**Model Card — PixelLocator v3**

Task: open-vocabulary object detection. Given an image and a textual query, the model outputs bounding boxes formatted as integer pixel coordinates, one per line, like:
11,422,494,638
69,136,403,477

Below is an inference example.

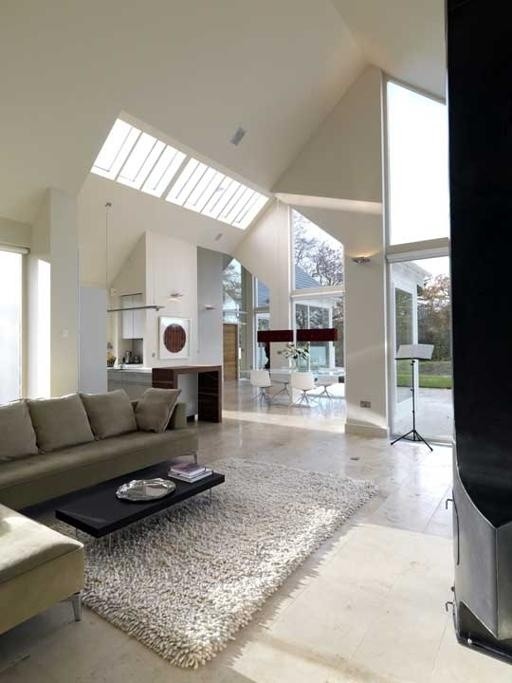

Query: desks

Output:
152,364,222,423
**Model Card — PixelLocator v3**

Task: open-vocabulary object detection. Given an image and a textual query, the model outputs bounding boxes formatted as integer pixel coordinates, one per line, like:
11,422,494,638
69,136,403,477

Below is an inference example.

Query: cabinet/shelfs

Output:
122,292,144,340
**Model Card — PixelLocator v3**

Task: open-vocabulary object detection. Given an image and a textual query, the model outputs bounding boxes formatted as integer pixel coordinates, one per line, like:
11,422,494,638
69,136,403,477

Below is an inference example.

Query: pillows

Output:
24,392,95,454
135,387,182,436
0,400,40,463
78,389,138,442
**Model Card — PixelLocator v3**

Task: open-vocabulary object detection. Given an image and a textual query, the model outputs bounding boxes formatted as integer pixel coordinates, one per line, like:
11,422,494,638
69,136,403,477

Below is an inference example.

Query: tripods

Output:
389,360,435,455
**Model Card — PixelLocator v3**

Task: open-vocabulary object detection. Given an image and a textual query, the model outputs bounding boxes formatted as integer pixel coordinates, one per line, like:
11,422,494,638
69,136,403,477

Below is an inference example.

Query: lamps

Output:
351,256,372,264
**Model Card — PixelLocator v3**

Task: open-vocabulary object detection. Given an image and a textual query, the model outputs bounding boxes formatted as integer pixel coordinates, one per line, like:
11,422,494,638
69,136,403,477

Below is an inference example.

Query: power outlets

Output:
360,400,371,409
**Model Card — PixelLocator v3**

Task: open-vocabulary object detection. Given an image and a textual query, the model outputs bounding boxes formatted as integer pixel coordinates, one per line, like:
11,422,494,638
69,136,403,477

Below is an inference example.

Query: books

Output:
168,461,214,483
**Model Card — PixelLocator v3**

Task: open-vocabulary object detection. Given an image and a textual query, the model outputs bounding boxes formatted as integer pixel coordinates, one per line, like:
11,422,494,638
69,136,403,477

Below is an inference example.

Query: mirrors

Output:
158,316,190,360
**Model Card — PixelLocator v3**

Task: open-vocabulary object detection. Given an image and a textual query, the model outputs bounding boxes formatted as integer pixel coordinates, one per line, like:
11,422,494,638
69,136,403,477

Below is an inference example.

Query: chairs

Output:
288,372,322,414
249,369,273,409
270,369,291,401
313,373,339,406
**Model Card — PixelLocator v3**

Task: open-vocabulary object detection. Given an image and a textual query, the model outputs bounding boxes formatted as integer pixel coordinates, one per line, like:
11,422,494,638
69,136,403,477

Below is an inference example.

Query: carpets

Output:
46,456,380,669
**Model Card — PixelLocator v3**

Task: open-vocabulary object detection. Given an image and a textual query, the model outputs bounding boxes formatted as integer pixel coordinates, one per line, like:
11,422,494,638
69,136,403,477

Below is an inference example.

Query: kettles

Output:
125,351,133,364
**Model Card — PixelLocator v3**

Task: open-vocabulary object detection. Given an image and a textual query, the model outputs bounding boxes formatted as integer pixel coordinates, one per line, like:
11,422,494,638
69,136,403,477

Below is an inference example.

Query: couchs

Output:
0,398,198,512
0,503,85,633
